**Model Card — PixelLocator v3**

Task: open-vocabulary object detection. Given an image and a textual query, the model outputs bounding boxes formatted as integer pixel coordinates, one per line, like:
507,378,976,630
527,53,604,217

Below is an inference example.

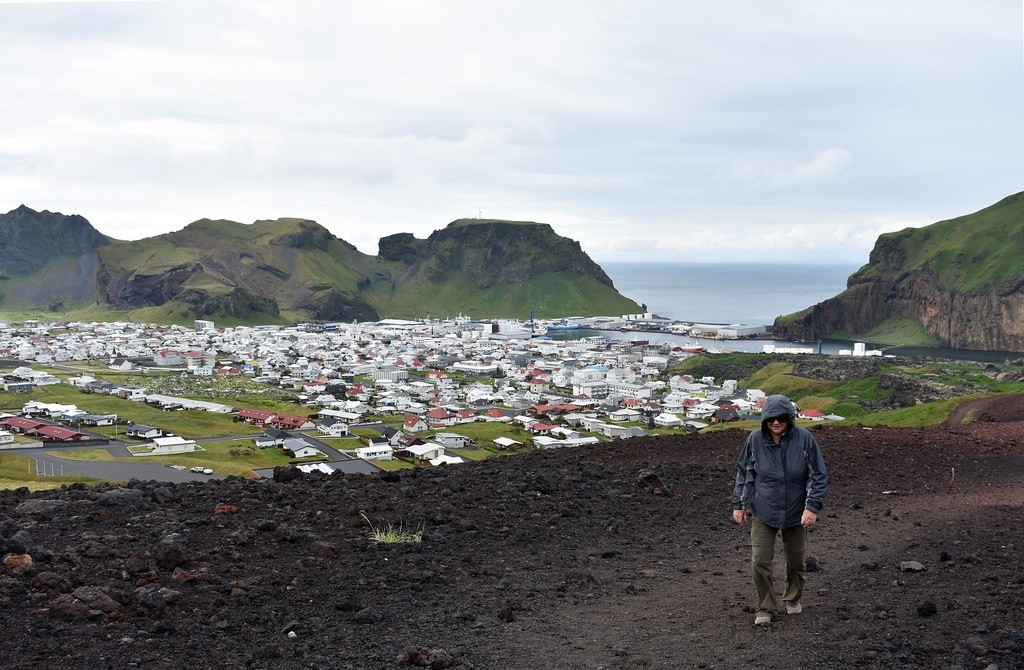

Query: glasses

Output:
765,415,790,424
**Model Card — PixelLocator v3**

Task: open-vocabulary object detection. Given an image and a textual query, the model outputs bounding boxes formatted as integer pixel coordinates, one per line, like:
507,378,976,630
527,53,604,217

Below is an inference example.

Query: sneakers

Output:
786,602,802,614
754,615,771,624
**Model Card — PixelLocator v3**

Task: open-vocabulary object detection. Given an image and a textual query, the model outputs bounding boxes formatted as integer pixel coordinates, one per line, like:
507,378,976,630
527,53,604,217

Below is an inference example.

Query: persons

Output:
732,393,829,625
